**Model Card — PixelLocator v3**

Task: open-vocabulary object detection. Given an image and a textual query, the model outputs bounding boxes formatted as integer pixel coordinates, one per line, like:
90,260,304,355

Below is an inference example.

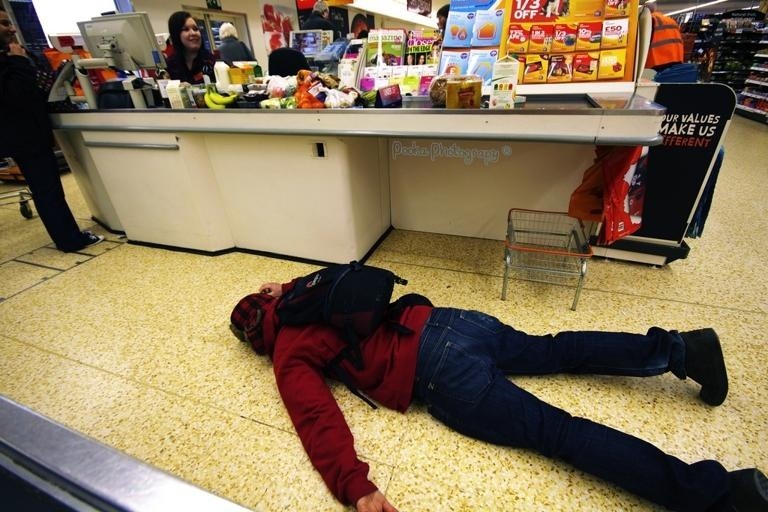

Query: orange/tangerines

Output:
294,84,325,108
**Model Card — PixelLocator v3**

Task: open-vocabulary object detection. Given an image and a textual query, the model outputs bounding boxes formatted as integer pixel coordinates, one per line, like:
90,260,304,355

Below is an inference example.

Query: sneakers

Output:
81,230,104,248
722,468,768,511
679,328,728,405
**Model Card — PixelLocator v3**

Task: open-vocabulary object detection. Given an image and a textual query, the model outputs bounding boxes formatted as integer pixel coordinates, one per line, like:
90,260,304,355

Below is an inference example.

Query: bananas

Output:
204,84,237,109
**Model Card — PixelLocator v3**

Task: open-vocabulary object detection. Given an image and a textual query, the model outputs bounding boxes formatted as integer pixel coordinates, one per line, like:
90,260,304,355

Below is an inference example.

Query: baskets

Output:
506,208,594,292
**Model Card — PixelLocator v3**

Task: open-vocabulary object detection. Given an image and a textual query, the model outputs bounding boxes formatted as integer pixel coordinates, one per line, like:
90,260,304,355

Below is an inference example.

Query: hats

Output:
231,293,276,356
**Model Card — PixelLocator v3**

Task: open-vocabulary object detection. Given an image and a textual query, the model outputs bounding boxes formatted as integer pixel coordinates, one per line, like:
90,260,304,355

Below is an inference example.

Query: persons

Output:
214,22,258,63
230,261,768,512
166,10,218,84
644,0,684,70
302,0,340,44
1,8,106,248
436,3,450,39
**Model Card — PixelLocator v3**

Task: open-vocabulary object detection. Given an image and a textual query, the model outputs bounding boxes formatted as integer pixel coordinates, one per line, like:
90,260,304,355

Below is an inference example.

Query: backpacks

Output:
269,261,394,363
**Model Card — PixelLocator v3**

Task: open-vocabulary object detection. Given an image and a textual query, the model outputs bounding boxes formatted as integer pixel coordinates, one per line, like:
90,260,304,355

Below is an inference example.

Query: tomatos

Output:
271,85,284,98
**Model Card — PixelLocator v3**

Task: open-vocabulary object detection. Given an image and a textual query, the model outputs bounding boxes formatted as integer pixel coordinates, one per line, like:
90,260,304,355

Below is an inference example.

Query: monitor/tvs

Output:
76,11,168,71
289,29,323,58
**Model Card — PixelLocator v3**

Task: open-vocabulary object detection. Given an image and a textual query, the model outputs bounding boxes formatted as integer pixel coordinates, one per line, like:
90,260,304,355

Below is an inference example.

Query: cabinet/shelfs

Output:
674,9,768,125
